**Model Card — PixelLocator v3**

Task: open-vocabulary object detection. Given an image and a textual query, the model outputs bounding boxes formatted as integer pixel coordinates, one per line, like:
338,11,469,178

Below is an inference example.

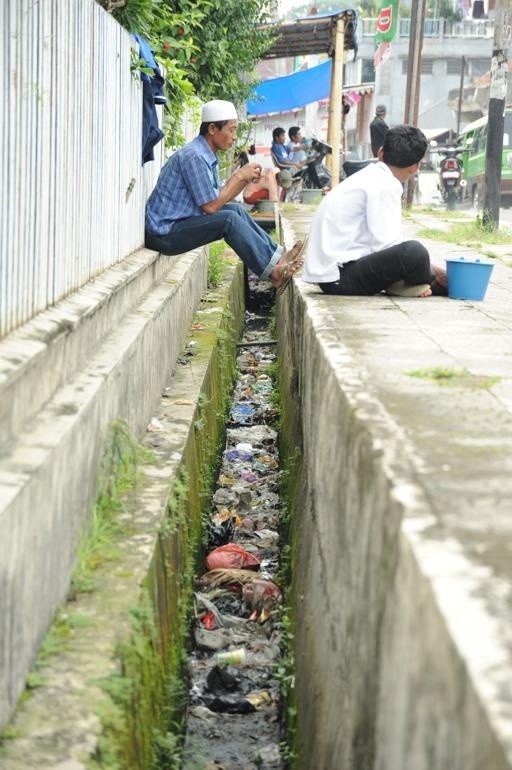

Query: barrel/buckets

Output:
301,188,323,205
255,200,276,214
446,257,495,301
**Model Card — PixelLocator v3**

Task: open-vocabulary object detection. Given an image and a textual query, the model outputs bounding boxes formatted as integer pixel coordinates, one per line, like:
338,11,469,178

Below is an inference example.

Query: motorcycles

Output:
432,143,470,210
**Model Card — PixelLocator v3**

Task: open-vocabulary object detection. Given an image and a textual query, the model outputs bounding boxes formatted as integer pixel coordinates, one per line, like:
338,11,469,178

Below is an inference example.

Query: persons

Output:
370,104,390,158
300,124,447,298
272,126,331,192
145,99,308,296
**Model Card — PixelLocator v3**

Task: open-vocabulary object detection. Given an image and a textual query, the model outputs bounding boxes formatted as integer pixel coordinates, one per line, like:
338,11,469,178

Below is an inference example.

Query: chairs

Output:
272,152,309,204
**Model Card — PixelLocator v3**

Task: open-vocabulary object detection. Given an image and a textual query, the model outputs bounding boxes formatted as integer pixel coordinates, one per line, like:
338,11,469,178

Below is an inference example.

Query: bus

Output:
455,105,512,210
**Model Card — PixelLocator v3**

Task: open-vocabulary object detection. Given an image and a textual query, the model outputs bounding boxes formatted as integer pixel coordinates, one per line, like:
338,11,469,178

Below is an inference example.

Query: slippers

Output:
275,259,305,296
285,234,309,263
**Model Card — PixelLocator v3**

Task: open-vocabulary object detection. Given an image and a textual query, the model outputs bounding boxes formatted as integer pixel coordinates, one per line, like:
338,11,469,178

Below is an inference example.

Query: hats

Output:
278,168,292,188
201,99,238,123
